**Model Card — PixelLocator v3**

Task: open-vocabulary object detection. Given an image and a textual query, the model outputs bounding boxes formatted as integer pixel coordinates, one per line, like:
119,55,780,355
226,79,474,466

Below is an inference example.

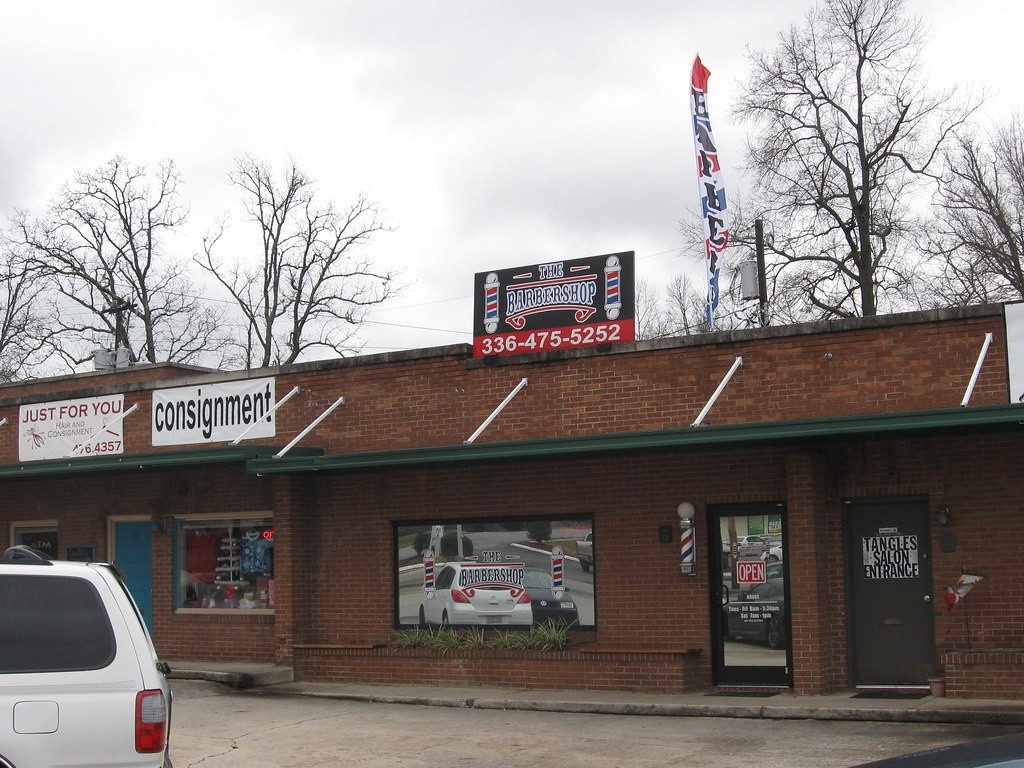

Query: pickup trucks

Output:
574,531,593,570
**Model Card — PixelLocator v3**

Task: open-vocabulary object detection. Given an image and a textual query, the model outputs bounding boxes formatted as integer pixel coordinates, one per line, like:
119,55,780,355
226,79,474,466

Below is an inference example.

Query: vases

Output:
927,675,946,697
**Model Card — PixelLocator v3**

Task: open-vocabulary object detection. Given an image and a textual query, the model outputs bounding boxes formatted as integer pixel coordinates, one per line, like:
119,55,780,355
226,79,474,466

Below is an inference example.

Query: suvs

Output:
0,544,174,768
418,562,534,627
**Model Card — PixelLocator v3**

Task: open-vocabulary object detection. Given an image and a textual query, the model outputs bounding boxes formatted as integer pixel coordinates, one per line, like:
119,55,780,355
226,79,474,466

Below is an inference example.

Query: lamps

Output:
677,502,697,576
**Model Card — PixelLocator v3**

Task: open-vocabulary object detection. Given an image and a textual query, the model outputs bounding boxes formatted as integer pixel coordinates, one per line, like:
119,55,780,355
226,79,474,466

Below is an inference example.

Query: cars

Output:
723,533,785,650
521,567,579,625
